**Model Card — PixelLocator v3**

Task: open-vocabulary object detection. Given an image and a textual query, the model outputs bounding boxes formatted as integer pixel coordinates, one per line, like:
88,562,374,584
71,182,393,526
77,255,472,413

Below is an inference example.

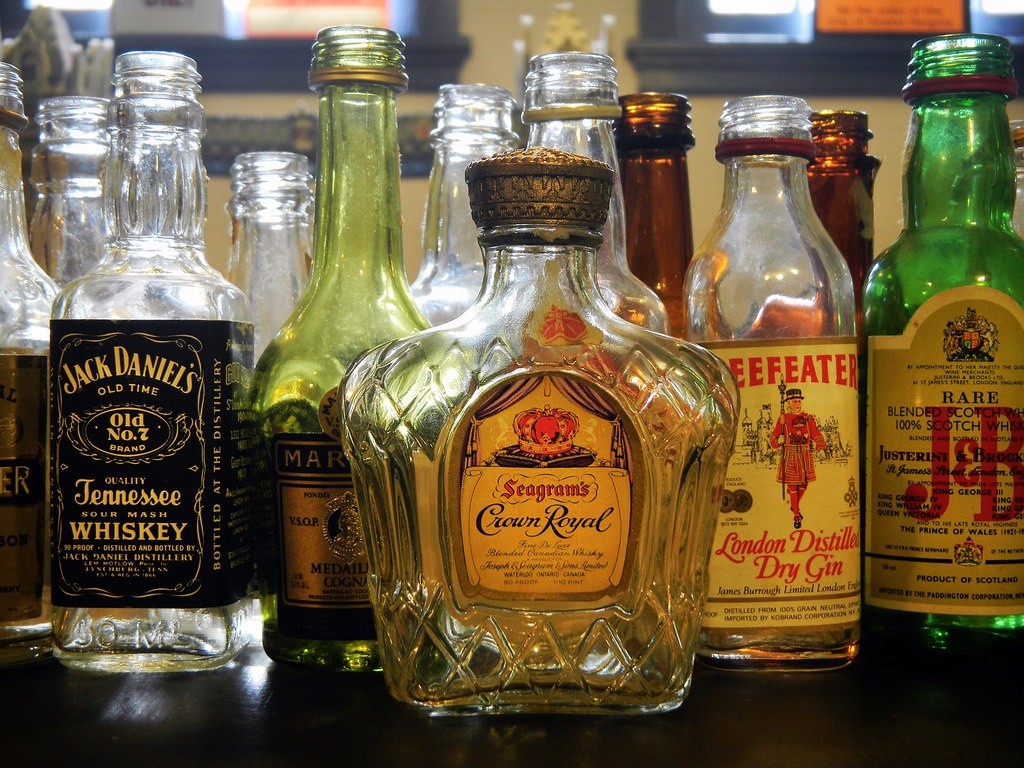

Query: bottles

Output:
813,111,883,296
525,51,669,339
1009,121,1024,240
0,61,62,643
336,154,739,711
249,29,431,672
25,101,125,284
45,51,251,674
680,95,863,666
406,86,522,330
228,150,317,362
619,92,694,337
861,34,1024,655
